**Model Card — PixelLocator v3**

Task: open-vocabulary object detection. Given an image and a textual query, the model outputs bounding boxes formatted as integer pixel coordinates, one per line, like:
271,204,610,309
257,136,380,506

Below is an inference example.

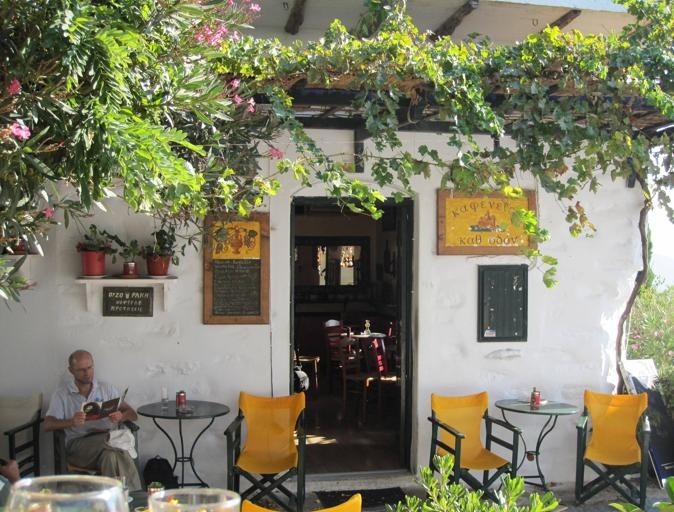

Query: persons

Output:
0,456,24,511
43,348,145,493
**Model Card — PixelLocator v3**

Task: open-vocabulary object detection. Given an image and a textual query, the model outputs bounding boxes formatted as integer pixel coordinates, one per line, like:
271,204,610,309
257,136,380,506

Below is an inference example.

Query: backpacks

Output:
143,455,179,489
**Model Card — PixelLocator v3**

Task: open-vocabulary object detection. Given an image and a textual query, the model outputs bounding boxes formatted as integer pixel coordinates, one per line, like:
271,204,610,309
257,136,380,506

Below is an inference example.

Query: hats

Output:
104,428,138,459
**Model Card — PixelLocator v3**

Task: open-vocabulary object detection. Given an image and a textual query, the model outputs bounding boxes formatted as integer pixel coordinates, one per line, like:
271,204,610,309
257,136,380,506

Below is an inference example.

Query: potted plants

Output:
74,230,177,278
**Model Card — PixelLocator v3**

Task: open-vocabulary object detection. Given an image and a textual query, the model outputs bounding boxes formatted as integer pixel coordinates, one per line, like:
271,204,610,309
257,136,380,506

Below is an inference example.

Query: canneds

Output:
530,391,541,411
176,391,186,409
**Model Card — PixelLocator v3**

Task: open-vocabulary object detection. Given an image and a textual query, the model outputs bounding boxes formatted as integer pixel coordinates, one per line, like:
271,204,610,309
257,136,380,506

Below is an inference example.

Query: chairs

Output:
425,388,651,510
320,318,400,425
0,390,43,477
222,390,307,512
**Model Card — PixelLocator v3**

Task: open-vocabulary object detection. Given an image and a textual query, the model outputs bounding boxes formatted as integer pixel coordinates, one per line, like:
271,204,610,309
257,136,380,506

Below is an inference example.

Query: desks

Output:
136,399,230,488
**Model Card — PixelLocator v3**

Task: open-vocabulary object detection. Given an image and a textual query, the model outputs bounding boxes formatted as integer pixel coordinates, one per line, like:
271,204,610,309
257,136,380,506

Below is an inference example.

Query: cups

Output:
529,390,541,410
160,383,168,402
174,390,187,410
4,473,131,512
146,487,241,512
